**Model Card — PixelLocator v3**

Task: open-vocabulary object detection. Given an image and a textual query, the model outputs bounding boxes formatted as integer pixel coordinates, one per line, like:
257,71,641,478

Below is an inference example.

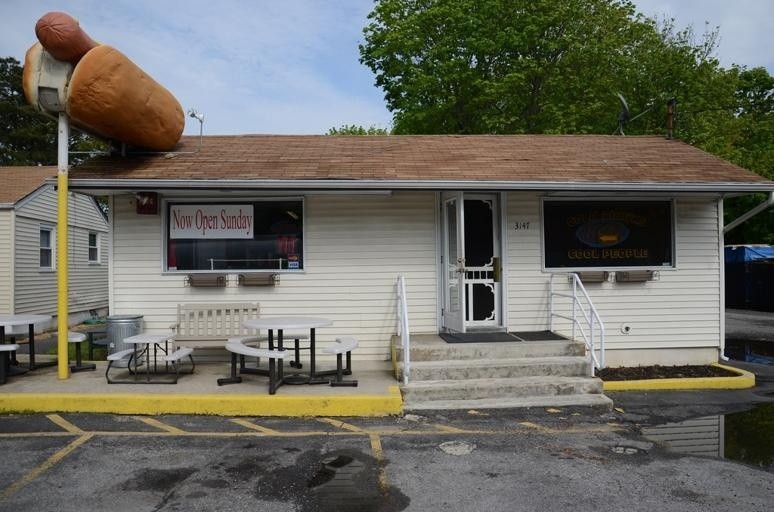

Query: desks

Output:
243,317,333,394
1,314,52,377
122,332,177,384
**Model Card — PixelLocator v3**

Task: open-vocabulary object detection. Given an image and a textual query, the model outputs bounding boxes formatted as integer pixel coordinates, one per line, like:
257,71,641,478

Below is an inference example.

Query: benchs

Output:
49,331,96,373
105,347,196,384
217,334,359,387
0,332,28,384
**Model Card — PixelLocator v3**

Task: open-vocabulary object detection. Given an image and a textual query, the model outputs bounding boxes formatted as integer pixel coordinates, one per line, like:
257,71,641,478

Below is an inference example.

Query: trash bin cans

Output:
105,314,144,368
